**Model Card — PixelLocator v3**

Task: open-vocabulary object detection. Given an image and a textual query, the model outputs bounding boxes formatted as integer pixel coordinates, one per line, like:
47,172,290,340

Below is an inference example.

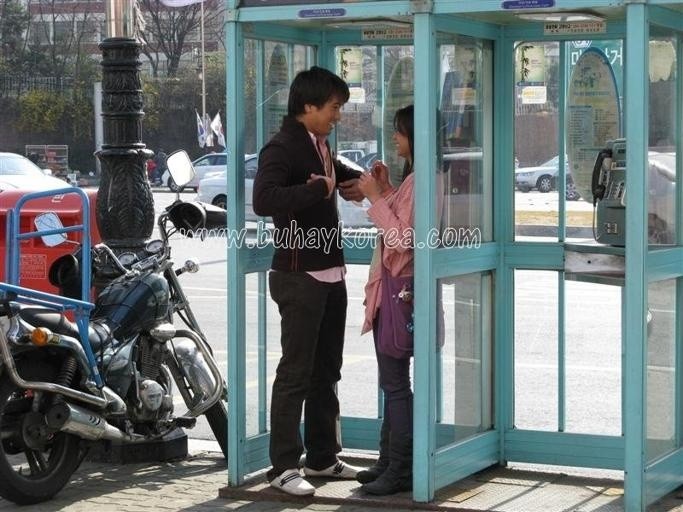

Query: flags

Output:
196,112,227,149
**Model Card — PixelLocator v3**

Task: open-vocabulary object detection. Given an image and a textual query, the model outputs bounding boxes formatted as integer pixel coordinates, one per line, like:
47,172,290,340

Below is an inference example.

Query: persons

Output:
28,150,39,166
253,65,370,497
152,147,168,186
144,158,157,183
356,102,451,496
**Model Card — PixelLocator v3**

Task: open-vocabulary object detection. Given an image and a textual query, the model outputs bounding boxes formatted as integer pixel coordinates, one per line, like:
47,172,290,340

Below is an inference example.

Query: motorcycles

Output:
0,146,229,506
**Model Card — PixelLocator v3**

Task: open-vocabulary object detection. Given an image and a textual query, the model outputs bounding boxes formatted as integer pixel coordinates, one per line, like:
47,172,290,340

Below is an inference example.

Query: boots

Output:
356,436,390,484
360,434,413,496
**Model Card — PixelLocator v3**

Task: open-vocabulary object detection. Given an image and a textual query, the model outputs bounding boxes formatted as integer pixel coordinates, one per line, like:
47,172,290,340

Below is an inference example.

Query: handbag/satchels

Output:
374,267,447,363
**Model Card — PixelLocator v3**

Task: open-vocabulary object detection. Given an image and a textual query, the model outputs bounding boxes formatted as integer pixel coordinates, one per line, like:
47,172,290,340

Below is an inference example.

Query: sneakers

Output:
301,454,369,481
269,466,317,499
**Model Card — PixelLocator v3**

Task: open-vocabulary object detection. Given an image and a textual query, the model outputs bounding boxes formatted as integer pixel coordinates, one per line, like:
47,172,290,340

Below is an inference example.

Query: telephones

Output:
591,138,626,245
